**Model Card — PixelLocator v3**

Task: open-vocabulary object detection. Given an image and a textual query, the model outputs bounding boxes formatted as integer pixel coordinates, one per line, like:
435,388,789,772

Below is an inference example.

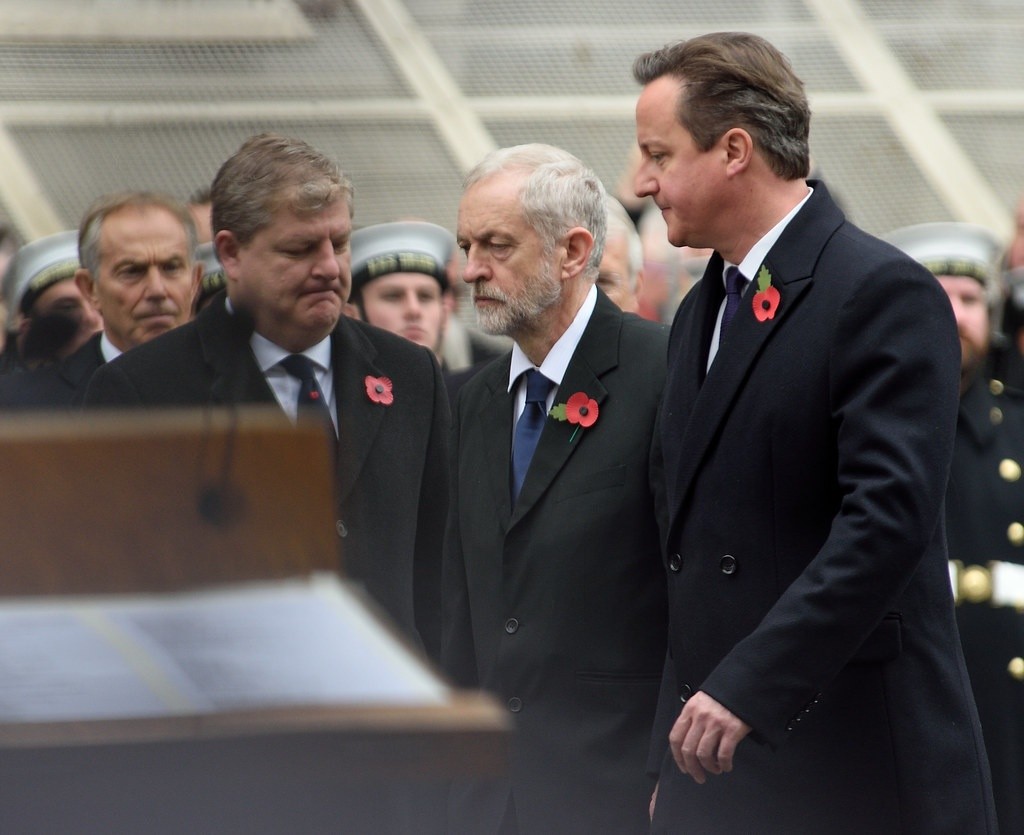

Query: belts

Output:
947,557,1023,610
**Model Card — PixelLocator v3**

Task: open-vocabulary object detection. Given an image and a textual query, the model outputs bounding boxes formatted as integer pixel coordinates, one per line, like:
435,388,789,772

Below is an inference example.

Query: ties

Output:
279,354,339,484
511,368,556,511
718,267,748,349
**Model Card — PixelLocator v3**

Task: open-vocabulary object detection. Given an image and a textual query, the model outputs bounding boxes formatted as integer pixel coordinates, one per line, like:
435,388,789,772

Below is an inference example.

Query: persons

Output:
1,179,1023,835
81,129,451,684
437,142,673,835
631,31,999,835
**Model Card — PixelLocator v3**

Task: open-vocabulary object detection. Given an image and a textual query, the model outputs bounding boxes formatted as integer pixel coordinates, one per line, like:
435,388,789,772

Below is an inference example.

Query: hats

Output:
2,230,80,318
881,222,1005,287
348,221,456,303
194,240,226,314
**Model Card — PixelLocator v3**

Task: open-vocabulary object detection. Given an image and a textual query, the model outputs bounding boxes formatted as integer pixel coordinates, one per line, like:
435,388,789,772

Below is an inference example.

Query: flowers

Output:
549,390,598,429
752,264,780,323
365,375,395,405
